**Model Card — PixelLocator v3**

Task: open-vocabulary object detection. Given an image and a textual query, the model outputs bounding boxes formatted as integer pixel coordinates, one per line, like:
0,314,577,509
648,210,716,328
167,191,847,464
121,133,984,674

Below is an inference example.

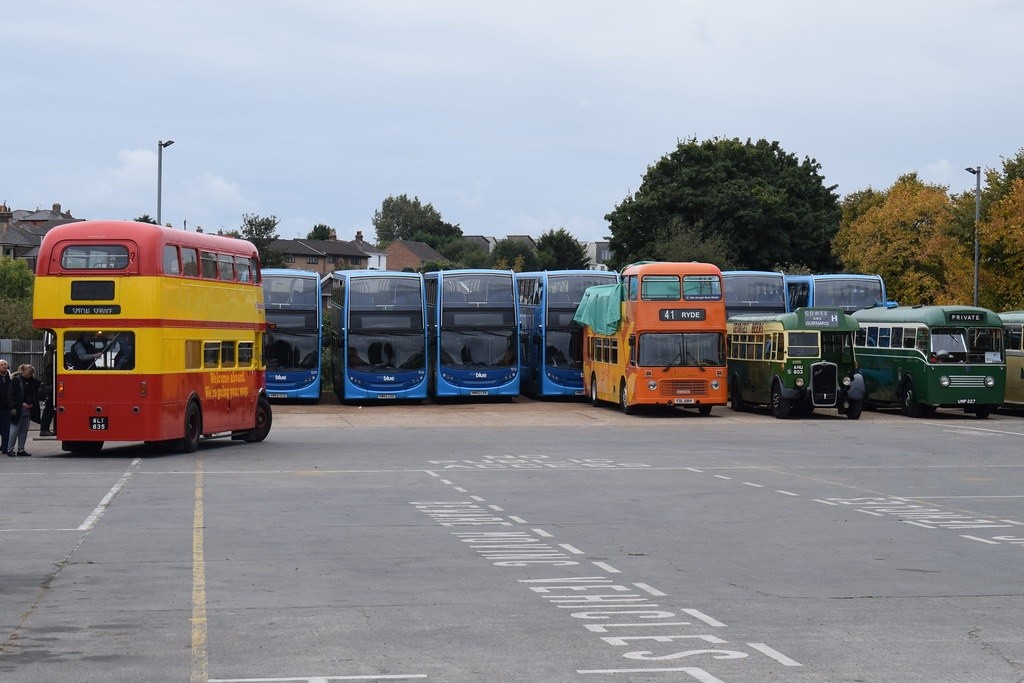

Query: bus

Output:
240,268,324,404
993,310,1024,412
577,260,728,417
330,268,428,405
423,269,520,403
852,304,1005,419
720,269,790,323
785,272,898,316
33,219,273,457
725,306,862,419
513,270,619,402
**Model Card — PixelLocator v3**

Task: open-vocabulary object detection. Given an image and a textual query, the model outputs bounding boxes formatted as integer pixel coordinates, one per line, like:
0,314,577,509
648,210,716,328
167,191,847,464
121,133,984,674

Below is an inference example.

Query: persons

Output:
0,359,12,454
7,363,41,457
65,344,77,369
114,334,134,369
71,333,111,369
39,333,66,436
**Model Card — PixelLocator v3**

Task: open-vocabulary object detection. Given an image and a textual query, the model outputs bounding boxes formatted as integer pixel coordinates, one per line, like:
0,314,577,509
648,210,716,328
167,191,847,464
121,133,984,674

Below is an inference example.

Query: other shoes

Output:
7,451,15,456
18,451,32,456
39,429,55,436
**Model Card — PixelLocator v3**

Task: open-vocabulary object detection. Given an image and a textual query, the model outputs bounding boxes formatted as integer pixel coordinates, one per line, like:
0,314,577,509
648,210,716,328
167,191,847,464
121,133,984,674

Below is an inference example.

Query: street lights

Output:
965,165,982,307
157,139,175,226
